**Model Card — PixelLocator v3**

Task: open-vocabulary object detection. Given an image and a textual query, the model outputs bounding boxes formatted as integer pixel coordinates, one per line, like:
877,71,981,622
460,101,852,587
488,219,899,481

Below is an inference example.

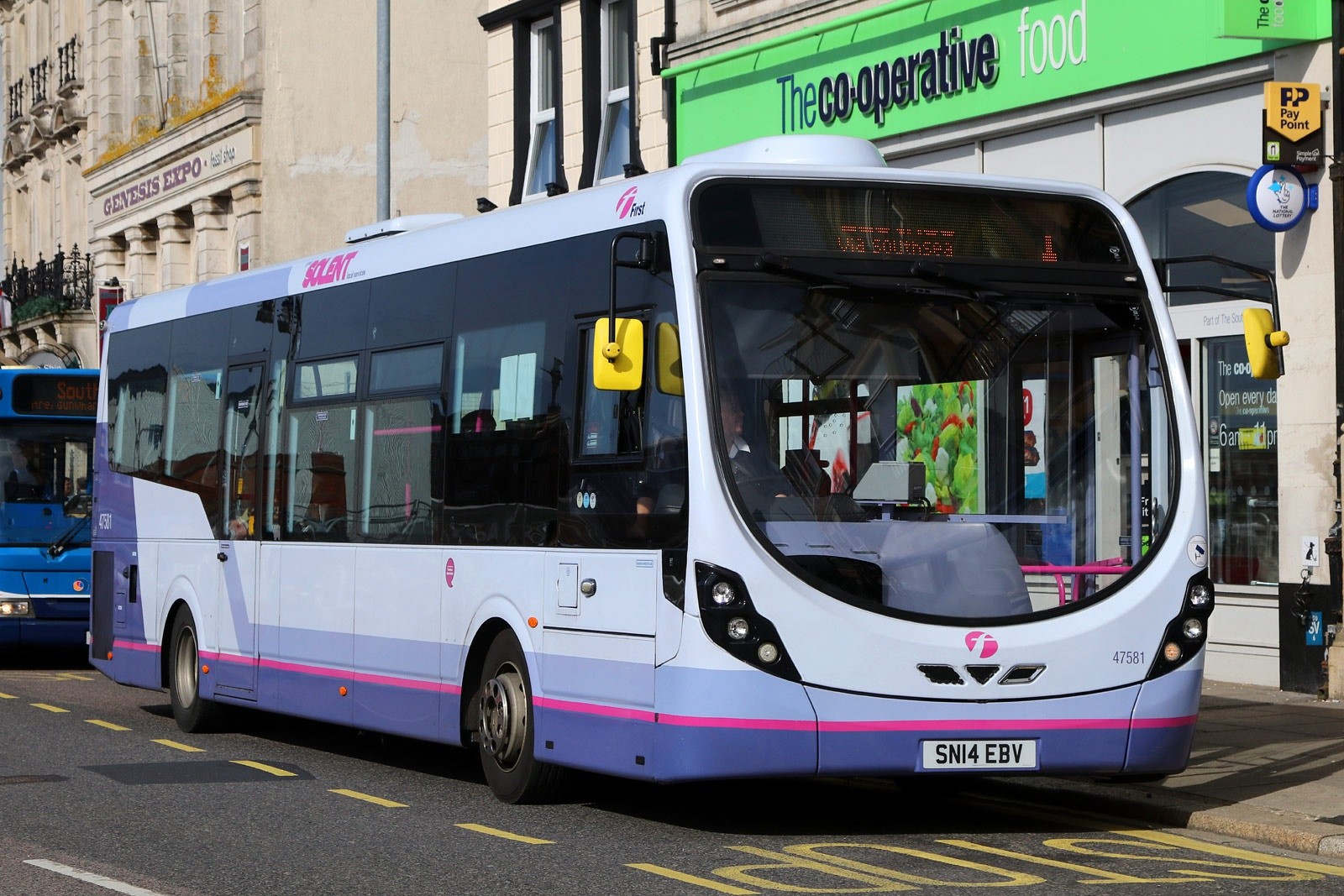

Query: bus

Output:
82,138,1295,802
0,362,101,667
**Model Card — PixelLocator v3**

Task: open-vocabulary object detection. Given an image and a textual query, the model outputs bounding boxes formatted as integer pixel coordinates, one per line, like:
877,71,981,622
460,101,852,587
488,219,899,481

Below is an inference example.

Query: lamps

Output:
545,182,569,197
623,164,648,178
476,198,498,213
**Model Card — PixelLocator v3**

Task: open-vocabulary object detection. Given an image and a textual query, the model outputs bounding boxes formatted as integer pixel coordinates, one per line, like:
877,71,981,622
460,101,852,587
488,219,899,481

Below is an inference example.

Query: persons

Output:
65,477,87,501
4,439,54,501
636,383,793,514
